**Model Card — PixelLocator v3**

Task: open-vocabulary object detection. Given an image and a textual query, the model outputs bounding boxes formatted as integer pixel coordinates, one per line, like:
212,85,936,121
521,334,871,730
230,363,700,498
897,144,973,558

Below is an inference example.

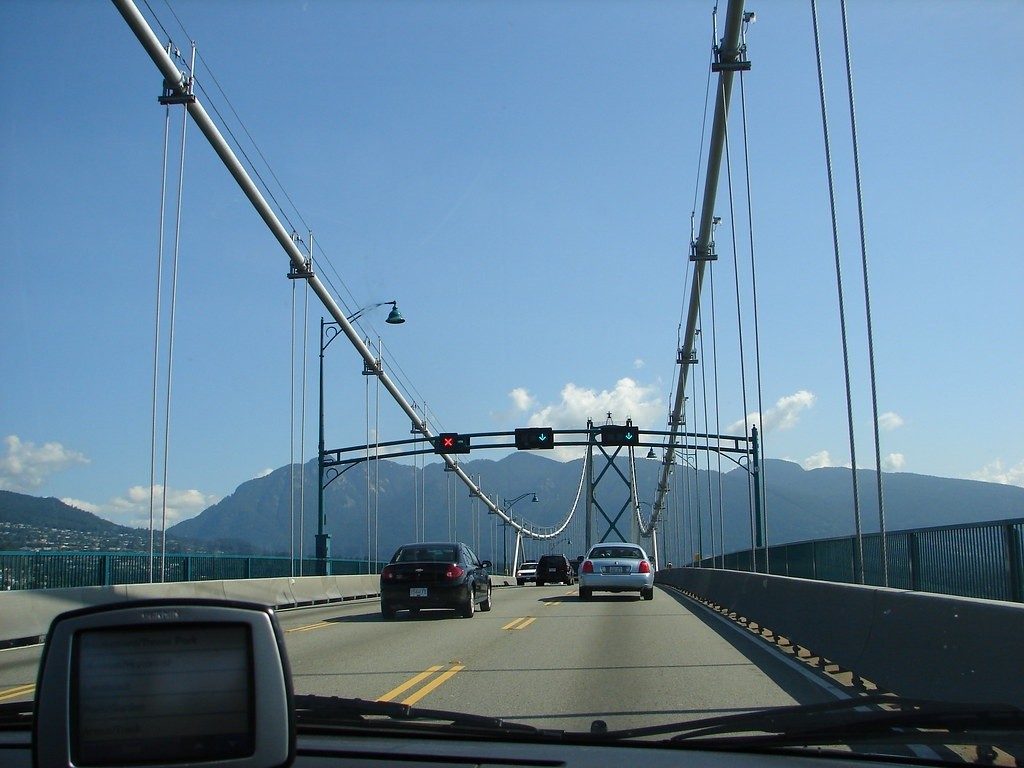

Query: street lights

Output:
647,443,703,568
315,299,405,576
504,492,540,576
548,538,572,556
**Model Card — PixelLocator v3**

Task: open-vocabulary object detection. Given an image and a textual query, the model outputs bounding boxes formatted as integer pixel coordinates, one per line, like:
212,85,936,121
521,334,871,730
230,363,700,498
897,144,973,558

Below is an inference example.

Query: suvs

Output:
536,553,574,587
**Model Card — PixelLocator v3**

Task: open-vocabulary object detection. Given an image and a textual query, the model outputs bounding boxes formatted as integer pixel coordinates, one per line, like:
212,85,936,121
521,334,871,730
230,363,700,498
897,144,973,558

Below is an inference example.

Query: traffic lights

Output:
602,426,639,446
434,433,471,454
515,428,554,450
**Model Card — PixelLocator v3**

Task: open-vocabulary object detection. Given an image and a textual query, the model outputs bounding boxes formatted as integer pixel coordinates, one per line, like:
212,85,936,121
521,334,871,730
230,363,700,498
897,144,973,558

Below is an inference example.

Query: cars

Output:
516,563,539,586
569,559,582,581
380,541,492,618
577,542,655,600
